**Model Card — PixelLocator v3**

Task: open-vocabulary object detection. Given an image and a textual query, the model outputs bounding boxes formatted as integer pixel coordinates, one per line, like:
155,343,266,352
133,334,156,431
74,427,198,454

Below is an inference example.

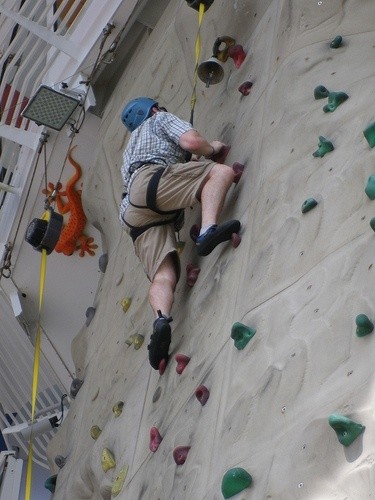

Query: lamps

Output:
21,85,81,134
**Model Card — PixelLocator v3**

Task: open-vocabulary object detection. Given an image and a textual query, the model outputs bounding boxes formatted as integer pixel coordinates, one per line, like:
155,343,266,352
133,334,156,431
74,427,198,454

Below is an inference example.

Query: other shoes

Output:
198,219,242,257
148,317,172,370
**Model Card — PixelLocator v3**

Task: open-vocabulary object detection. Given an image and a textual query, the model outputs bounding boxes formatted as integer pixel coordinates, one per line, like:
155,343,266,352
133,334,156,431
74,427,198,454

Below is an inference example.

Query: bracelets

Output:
204,145,215,158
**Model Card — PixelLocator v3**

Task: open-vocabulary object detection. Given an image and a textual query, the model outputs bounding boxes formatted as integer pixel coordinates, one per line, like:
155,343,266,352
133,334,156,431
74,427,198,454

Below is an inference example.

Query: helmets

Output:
120,97,159,132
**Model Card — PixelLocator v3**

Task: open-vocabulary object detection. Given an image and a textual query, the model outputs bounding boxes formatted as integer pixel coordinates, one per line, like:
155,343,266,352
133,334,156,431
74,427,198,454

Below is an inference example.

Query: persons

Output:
118,97,240,370
